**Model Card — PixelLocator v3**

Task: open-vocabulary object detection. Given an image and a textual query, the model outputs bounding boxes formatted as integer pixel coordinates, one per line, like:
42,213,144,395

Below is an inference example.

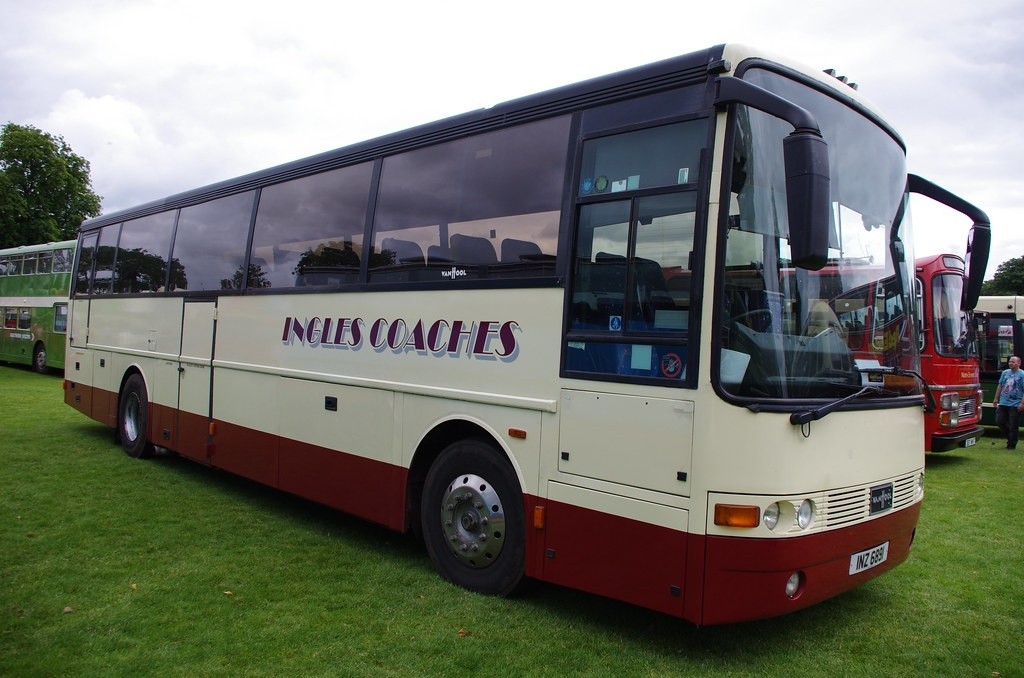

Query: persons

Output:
993,355,1024,451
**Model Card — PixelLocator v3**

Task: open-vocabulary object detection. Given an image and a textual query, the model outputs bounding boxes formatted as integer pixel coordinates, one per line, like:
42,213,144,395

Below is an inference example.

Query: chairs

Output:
596,252,675,321
381,238,424,266
253,256,272,273
428,245,450,258
501,238,542,261
450,234,497,264
273,247,303,286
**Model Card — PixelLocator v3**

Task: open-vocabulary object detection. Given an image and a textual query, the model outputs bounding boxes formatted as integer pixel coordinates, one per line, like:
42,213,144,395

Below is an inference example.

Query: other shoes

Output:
1006,439,1018,449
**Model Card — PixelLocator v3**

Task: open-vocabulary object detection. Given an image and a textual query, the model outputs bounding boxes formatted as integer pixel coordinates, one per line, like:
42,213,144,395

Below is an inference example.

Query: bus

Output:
839,294,1024,429
62,42,994,626
662,253,991,453
0,238,79,377
